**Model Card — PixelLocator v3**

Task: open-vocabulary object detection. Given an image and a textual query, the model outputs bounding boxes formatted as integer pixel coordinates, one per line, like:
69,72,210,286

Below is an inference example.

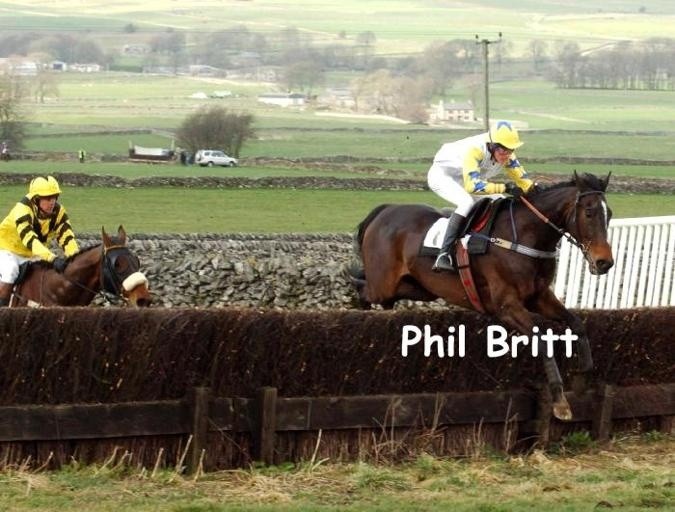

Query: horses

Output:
339,170,614,421
6,225,152,308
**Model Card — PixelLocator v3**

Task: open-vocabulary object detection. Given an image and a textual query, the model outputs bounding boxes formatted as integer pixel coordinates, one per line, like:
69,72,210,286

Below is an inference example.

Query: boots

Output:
432,213,465,271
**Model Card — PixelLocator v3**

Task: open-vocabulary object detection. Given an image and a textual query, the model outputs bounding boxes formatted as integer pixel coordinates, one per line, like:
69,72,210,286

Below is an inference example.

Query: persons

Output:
427,120,534,273
181,150,187,165
78,147,86,163
0,175,81,307
2,145,10,161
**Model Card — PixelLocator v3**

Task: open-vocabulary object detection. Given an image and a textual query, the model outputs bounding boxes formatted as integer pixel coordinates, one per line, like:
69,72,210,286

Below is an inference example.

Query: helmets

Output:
485,121,523,149
29,175,62,197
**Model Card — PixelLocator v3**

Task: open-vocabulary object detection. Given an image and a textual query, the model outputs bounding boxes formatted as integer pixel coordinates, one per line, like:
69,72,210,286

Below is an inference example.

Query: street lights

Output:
475,30,503,134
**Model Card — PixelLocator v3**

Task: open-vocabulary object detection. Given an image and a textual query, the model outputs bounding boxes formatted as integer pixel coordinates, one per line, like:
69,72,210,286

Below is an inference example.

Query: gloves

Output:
52,256,67,272
504,182,524,195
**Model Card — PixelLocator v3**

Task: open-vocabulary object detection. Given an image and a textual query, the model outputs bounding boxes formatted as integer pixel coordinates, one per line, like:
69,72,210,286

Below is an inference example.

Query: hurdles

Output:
0,306,673,470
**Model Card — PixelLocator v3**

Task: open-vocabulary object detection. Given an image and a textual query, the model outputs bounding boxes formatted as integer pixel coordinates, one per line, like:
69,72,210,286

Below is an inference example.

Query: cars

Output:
195,149,239,168
